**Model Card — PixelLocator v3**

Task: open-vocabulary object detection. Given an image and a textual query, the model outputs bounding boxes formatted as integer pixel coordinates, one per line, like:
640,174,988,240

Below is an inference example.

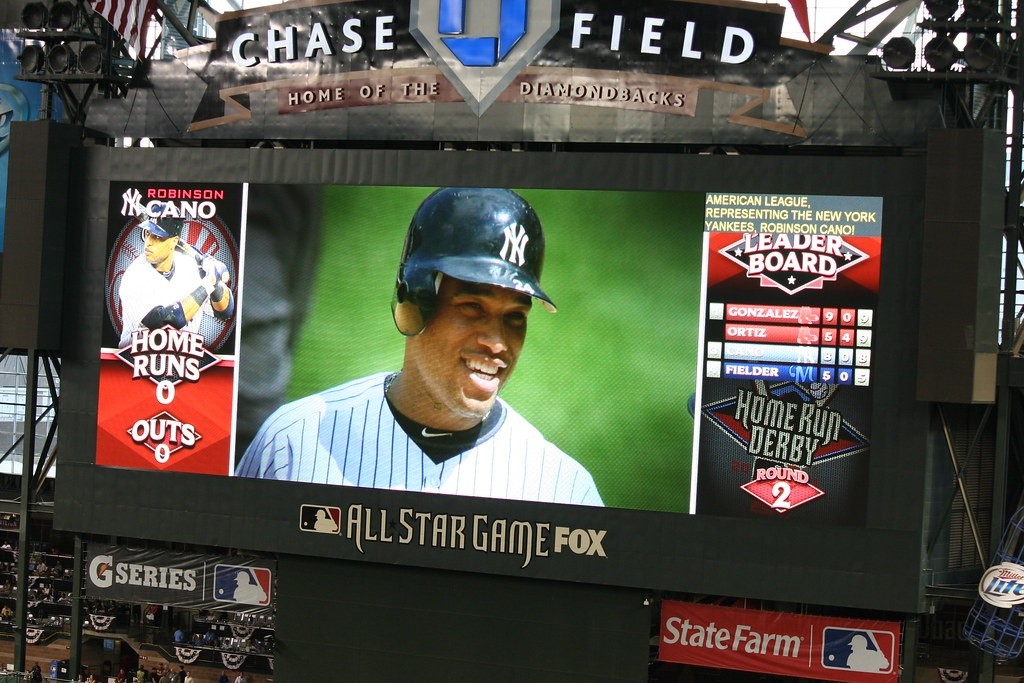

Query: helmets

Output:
390,187,557,334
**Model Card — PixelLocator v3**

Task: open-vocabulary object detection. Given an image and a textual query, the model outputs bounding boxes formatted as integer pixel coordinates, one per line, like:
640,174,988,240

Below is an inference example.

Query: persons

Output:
0,534,270,683
119,204,235,349
232,187,607,507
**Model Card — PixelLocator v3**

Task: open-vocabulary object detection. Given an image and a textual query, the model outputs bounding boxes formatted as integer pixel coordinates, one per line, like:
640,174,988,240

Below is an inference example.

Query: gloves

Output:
194,255,225,302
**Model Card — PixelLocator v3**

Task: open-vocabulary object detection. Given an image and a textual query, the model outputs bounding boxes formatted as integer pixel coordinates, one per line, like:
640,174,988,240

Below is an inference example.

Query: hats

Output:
138,202,185,239
